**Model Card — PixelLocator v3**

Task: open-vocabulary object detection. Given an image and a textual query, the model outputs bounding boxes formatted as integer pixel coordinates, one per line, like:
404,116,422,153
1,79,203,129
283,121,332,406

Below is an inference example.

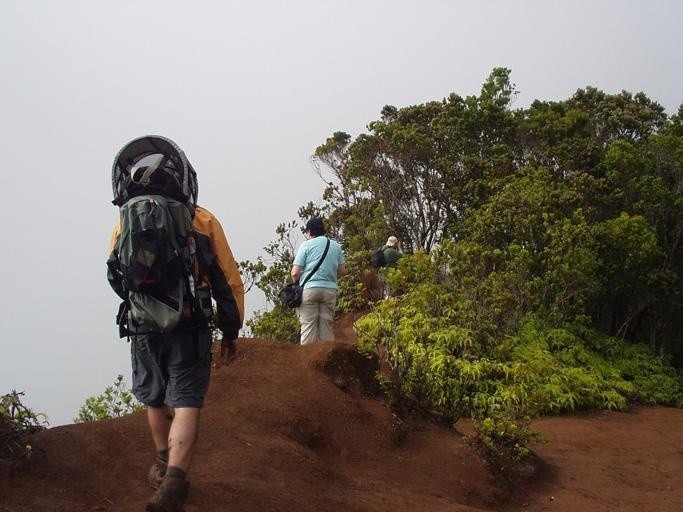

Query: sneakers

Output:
148,460,167,489
147,477,190,512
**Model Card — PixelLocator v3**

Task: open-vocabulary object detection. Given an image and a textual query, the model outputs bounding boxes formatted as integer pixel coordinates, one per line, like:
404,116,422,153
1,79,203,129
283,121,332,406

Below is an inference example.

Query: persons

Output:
289,217,347,347
375,235,405,302
104,154,244,510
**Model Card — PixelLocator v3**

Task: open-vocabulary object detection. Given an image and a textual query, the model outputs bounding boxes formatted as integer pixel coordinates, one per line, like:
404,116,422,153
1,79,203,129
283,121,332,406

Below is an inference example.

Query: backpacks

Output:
107,195,214,338
371,247,390,268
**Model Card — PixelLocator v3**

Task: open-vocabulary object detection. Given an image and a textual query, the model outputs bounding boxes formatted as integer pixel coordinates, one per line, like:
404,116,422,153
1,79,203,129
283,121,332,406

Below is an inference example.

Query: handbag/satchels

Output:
278,283,302,308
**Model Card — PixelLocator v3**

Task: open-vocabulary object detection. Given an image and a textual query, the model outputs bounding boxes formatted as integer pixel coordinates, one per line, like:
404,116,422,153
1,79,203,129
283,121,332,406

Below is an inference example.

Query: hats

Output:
386,236,398,247
